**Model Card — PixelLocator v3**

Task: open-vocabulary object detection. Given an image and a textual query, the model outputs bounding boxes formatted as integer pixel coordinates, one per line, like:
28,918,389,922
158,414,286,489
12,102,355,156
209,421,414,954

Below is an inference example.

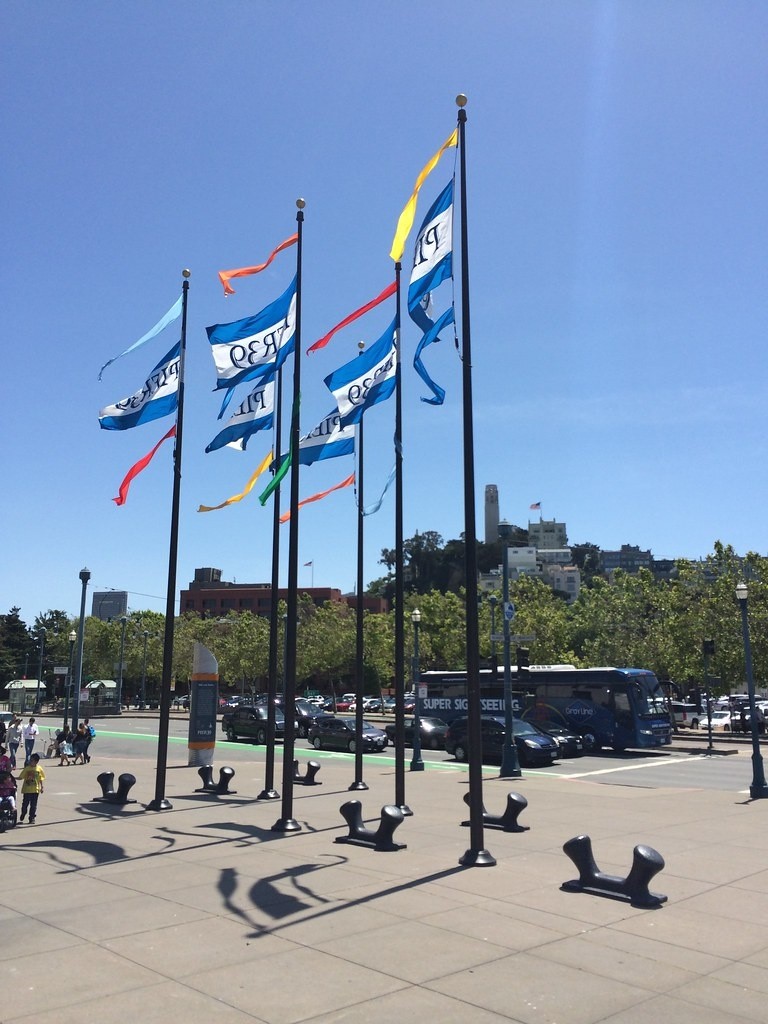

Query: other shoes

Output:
67,761,71,766
58,763,63,766
44,754,51,759
20,814,25,821
87,755,91,763
72,760,76,765
28,814,36,818
12,765,16,770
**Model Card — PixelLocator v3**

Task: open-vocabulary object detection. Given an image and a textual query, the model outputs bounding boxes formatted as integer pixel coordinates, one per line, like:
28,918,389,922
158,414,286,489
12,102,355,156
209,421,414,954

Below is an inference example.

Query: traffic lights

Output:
517,646,530,679
702,639,714,655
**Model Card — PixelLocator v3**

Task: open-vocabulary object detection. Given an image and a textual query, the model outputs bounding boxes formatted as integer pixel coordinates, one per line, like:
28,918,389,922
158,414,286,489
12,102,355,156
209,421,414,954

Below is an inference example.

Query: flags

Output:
203,274,299,393
529,501,541,510
98,340,183,430
323,316,398,431
269,406,355,474
408,176,455,343
205,368,275,452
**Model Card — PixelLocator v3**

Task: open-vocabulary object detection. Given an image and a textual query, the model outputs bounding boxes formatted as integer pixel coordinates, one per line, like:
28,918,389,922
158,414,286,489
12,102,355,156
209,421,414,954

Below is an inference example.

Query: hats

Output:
14,717,23,722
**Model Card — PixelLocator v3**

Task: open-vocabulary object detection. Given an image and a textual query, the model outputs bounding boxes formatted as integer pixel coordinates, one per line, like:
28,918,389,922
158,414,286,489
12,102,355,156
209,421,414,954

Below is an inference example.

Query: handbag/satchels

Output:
65,729,78,743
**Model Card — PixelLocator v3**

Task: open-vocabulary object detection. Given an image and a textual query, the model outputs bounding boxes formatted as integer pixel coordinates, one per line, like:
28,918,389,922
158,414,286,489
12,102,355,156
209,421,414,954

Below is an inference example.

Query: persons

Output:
52,695,60,710
14,753,45,824
45,718,92,766
125,694,140,710
740,705,765,734
22,717,39,766
5,719,23,771
0,713,20,744
0,746,17,824
93,693,98,706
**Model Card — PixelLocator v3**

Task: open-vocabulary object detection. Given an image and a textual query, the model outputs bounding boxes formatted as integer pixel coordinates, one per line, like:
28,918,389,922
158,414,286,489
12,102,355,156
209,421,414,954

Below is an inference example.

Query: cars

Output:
649,692,768,733
386,717,451,749
220,704,298,743
446,715,559,767
276,702,335,738
175,690,415,714
307,717,389,752
521,716,585,759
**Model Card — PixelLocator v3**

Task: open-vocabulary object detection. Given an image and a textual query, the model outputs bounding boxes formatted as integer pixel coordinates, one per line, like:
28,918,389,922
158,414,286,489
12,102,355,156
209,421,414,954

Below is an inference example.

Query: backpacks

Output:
86,725,96,737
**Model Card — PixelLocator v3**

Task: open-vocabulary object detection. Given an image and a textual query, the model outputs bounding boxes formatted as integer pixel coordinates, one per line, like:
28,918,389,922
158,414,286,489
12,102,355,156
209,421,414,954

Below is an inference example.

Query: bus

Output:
423,664,681,754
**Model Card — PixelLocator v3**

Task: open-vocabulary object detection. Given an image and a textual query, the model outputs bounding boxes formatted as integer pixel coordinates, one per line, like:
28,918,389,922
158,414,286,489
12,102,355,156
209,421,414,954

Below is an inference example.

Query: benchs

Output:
731,719,765,734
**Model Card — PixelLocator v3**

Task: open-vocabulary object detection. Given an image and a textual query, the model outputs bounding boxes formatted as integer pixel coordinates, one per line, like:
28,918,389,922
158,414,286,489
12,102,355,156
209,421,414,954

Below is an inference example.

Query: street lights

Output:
72,567,92,732
138,630,149,711
490,592,498,671
115,614,126,715
32,626,47,714
63,628,76,730
24,653,30,679
735,578,768,797
410,607,424,770
